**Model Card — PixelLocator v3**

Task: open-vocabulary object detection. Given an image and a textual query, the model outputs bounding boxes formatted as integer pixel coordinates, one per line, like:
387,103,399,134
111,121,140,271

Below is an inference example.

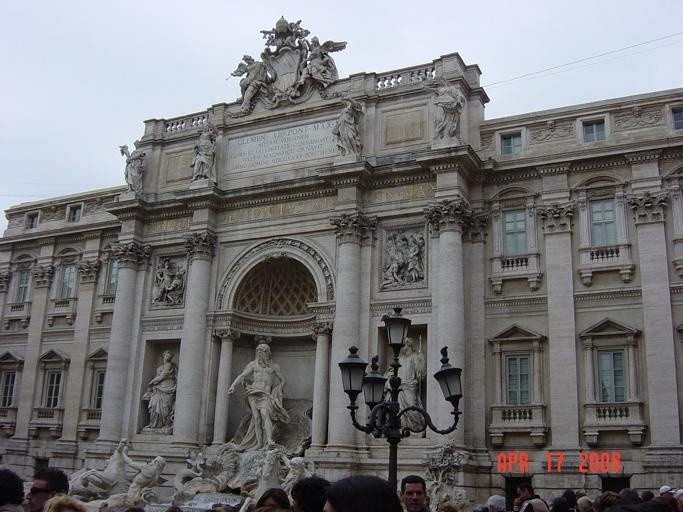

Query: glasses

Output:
29,487,49,494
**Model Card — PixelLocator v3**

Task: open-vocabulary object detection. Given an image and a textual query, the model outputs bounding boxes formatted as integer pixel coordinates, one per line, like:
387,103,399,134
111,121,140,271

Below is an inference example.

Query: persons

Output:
76,434,130,496
284,456,313,492
328,96,363,153
226,342,288,451
144,349,178,431
189,121,218,181
295,36,327,92
403,237,419,282
231,56,267,111
380,255,403,288
115,440,167,498
165,265,185,302
422,81,467,139
156,257,176,303
119,139,144,194
382,342,428,437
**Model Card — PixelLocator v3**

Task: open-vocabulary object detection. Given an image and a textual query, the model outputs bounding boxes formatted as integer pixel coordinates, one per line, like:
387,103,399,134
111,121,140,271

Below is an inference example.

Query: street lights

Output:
337,307,464,487
